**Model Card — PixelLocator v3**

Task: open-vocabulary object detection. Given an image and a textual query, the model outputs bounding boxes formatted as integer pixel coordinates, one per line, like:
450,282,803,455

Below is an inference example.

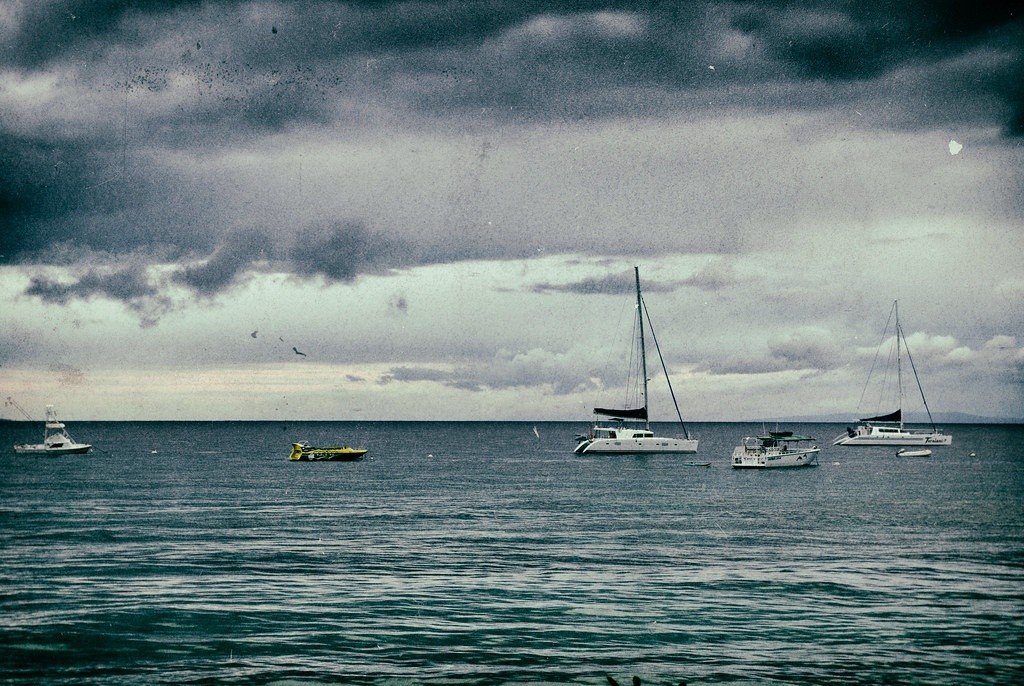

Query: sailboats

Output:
832,296,953,447
573,263,700,456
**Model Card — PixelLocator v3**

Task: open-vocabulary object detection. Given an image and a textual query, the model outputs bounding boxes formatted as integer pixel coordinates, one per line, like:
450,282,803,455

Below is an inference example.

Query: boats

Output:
287,439,369,462
730,416,822,470
896,448,933,458
680,460,712,466
4,395,96,456
